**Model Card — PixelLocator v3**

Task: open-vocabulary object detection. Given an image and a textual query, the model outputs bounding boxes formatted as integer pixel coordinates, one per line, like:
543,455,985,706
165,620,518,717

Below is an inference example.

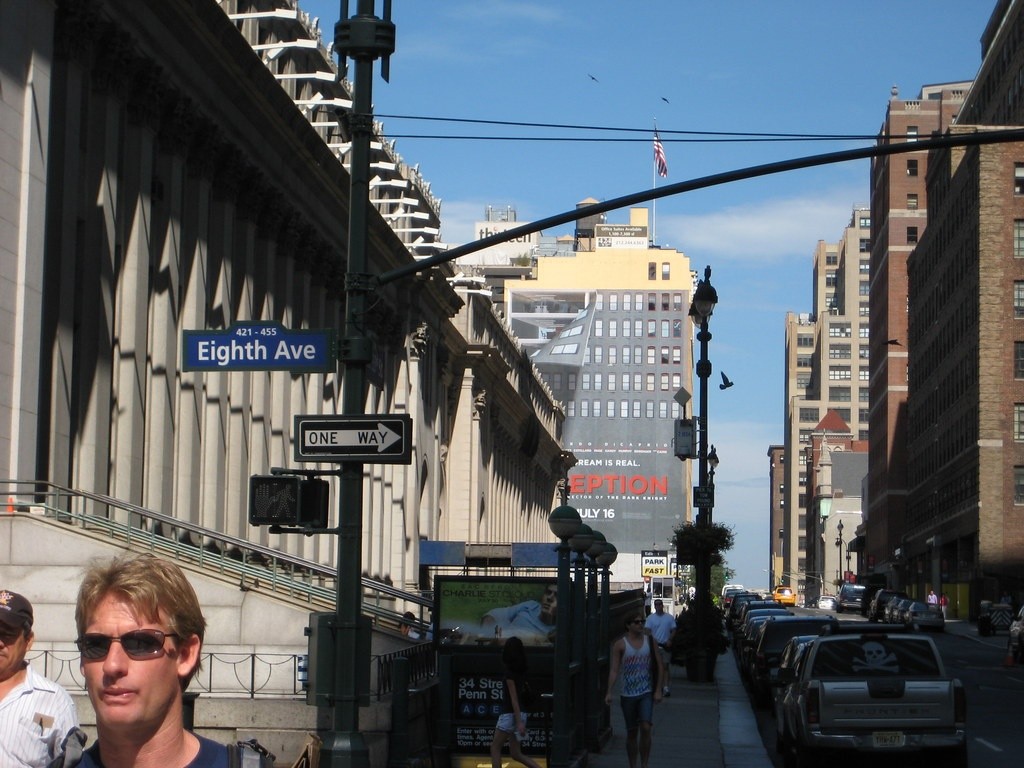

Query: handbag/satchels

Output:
648,635,659,690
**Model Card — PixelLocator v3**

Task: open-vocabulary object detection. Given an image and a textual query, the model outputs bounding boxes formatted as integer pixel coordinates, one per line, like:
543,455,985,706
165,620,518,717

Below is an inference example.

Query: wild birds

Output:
719,371,734,390
588,74,599,82
883,339,902,347
661,97,669,104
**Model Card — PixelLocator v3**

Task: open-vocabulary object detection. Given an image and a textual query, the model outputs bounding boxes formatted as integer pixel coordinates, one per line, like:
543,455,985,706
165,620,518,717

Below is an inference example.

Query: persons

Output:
606,616,664,768
473,584,563,647
398,613,423,641
46,553,275,768
928,591,937,612
490,636,540,768
0,589,81,768
643,600,676,697
939,594,949,622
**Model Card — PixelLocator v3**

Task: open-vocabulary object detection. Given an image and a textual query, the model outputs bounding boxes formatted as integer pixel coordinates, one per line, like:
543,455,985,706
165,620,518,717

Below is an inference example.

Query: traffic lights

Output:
248,474,301,528
301,479,330,529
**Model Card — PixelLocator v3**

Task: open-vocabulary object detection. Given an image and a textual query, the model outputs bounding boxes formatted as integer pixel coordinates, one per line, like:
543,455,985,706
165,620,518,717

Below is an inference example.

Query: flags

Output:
653,123,669,177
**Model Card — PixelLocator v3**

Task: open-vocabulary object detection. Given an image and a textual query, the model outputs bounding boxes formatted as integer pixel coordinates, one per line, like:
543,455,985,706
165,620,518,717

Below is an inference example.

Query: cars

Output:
900,602,945,632
1007,606,1024,664
816,583,866,613
744,615,840,694
774,635,821,754
721,585,796,677
860,585,916,624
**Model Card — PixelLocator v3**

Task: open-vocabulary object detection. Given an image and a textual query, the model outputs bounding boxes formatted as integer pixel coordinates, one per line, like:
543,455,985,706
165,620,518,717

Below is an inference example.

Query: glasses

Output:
655,603,663,605
631,619,646,626
74,629,179,659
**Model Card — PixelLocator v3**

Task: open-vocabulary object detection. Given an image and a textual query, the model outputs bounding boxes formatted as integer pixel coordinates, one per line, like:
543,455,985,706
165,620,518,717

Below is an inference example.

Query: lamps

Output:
449,272,466,287
381,212,430,221
342,162,397,171
274,70,337,82
293,97,353,108
334,141,353,159
251,39,320,52
368,179,408,188
392,226,439,235
369,175,382,191
407,237,425,251
262,40,287,68
371,197,419,206
413,254,455,262
404,242,448,250
302,92,323,116
446,277,486,283
455,289,493,297
386,208,404,225
328,140,383,151
228,9,302,22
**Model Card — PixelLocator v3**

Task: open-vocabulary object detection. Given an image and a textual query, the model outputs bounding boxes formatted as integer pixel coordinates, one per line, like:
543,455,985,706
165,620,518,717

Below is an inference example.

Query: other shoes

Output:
662,686,670,697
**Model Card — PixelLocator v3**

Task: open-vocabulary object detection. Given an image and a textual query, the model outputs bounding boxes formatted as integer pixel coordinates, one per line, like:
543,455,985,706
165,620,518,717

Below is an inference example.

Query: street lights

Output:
567,524,596,664
548,505,582,768
707,445,719,526
687,266,718,683
586,530,607,750
596,543,612,738
837,520,843,589
835,538,849,571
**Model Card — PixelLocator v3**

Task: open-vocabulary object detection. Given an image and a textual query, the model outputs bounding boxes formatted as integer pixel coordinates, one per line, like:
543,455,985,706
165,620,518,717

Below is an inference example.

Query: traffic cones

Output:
1005,644,1014,666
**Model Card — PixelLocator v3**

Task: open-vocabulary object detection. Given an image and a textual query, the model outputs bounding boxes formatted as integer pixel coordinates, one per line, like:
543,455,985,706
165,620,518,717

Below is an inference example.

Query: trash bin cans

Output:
978,614,992,637
182,692,200,731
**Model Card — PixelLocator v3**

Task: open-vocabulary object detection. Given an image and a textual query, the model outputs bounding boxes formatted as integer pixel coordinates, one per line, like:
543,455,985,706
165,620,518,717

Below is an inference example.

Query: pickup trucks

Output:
776,623,968,768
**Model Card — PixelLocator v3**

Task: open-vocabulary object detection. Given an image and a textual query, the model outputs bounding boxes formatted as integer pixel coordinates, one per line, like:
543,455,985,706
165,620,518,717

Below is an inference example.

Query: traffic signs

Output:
294,415,409,463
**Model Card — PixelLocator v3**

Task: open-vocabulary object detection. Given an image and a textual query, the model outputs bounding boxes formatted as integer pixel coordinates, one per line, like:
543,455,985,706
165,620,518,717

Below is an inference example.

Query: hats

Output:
0,590,34,633
